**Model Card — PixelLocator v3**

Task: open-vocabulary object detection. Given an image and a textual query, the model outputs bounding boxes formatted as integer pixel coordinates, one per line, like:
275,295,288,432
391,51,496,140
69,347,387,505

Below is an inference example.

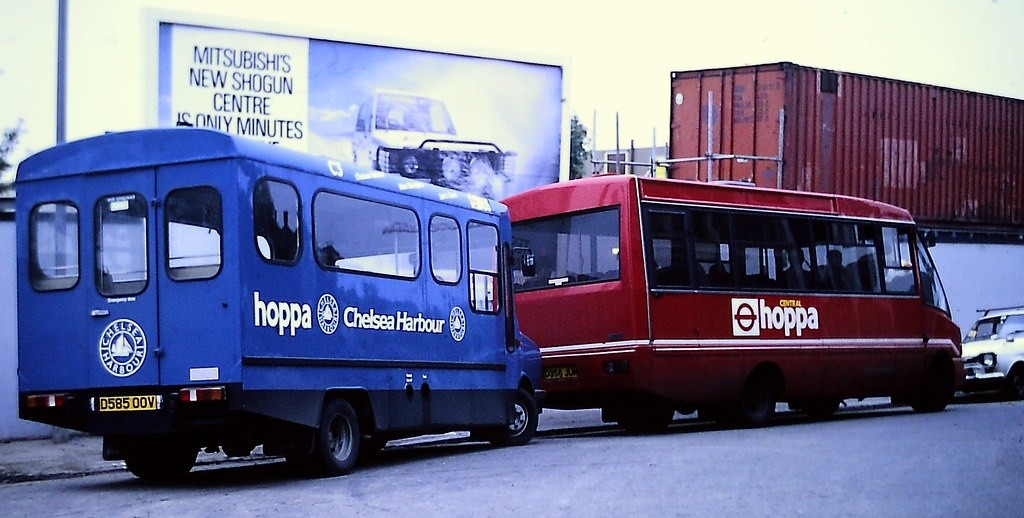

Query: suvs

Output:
351,88,508,193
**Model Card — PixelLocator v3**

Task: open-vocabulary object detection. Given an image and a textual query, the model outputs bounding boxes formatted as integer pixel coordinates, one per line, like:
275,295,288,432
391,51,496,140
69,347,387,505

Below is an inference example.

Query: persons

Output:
814,250,858,283
778,249,817,289
708,262,728,275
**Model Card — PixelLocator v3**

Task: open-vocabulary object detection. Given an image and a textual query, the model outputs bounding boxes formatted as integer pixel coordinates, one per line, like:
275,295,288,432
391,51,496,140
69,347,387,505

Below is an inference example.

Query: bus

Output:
12,122,546,478
491,171,968,434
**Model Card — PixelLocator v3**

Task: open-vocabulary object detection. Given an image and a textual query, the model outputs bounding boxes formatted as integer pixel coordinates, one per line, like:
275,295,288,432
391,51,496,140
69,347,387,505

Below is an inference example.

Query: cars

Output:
959,310,1024,400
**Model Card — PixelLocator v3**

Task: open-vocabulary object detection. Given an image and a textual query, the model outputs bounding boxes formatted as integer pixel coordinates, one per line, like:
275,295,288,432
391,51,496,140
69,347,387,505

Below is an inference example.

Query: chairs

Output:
655,252,877,293
